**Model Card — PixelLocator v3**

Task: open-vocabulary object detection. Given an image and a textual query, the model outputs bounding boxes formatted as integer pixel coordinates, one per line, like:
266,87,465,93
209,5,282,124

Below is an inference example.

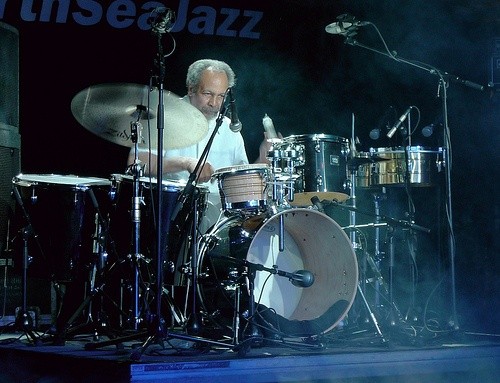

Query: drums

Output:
214,163,269,214
11,173,111,284
357,144,444,189
111,173,210,287
271,133,350,206
195,207,359,337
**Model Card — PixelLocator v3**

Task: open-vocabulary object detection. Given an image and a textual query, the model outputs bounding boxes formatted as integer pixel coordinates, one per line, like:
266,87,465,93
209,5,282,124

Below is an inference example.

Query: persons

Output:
128,60,283,258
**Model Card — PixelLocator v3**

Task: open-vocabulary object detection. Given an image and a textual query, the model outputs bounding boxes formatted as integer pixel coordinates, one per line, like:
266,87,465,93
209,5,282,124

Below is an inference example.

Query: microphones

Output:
325,20,370,34
229,90,243,132
150,7,175,33
387,106,412,139
291,269,313,288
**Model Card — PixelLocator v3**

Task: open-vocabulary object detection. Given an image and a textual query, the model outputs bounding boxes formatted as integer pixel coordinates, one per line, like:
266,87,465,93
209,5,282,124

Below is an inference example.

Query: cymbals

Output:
70,84,209,150
325,20,353,34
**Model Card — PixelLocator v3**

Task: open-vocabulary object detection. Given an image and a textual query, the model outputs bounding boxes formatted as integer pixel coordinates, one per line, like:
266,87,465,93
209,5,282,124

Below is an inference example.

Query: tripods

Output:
0,33,324,358
